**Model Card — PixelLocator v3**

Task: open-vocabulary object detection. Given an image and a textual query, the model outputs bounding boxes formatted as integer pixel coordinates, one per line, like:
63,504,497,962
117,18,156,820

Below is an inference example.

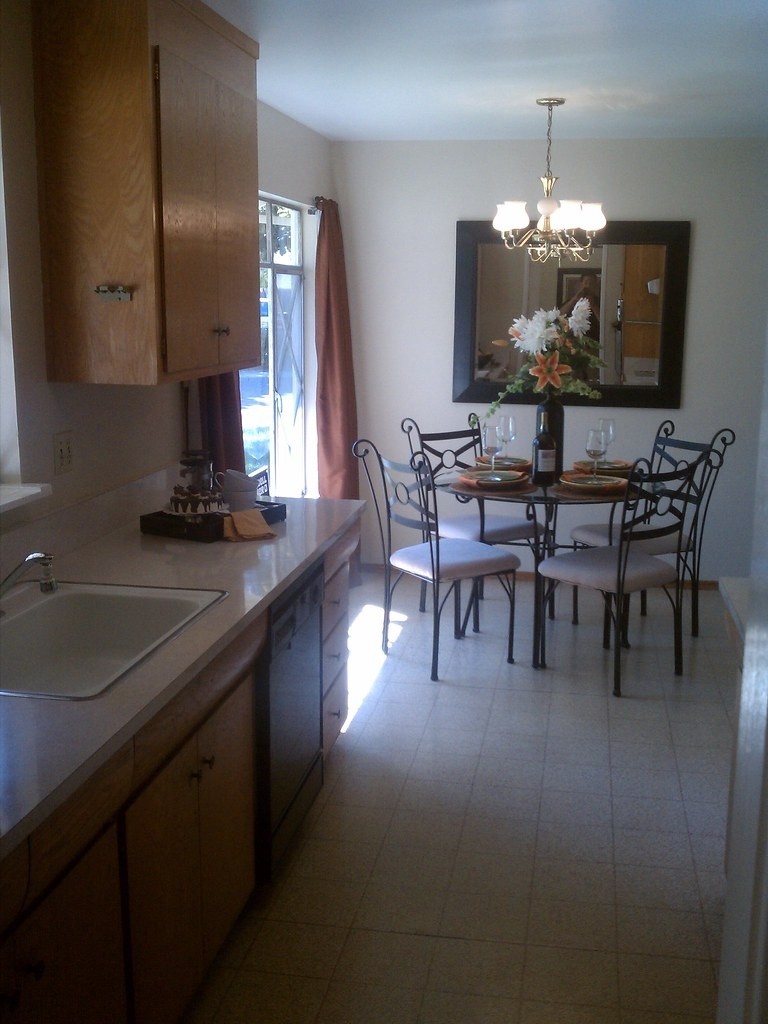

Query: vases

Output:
535,386,564,482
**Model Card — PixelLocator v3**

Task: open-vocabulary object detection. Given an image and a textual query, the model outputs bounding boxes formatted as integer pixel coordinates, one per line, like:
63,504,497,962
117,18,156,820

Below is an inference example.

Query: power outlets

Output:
54,431,78,474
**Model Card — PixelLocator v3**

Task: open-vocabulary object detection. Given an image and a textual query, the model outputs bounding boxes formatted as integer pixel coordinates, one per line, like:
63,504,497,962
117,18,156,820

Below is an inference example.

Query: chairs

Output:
351,412,736,696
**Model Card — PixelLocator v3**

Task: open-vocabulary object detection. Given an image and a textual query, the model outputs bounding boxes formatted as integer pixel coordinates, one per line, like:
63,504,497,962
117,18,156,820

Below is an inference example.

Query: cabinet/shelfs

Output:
24,0,261,384
0,516,365,1024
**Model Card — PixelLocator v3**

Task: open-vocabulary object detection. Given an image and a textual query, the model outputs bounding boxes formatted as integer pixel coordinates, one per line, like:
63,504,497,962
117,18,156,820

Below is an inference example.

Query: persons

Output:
558,272,600,379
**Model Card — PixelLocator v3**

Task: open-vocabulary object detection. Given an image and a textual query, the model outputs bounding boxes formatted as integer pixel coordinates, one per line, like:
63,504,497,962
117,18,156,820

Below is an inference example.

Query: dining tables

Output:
434,465,665,669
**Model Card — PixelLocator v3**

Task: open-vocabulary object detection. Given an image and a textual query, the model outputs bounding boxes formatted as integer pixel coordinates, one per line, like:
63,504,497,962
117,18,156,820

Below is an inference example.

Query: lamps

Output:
492,98,607,267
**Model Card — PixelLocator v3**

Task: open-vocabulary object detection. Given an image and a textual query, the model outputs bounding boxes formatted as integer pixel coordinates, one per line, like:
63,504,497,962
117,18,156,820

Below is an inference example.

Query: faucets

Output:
0,551,56,617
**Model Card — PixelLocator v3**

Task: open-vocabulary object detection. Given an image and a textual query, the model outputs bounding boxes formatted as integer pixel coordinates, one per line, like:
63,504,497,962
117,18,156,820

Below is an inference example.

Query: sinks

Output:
0,581,230,702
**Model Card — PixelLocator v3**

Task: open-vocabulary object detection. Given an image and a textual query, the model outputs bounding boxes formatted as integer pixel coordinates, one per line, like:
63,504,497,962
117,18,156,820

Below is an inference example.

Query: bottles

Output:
532,412,557,487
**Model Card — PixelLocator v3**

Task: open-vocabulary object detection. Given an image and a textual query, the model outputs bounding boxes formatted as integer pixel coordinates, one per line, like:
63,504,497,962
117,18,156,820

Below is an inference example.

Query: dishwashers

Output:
252,559,325,886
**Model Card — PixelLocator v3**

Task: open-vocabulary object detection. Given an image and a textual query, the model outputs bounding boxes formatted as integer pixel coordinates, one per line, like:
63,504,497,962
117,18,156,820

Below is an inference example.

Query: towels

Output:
223,509,277,542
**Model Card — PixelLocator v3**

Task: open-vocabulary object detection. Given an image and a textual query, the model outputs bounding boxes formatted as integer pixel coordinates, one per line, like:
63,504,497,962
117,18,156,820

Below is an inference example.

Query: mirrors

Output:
452,221,691,409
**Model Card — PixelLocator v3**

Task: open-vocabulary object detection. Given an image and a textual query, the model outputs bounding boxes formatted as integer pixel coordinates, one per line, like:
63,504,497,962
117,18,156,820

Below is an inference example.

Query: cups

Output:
215,468,259,512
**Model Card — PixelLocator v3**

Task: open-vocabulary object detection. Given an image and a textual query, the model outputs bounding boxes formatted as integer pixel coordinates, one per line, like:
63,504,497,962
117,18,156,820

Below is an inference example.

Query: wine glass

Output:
595,419,616,468
495,415,517,465
584,429,608,485
480,426,503,482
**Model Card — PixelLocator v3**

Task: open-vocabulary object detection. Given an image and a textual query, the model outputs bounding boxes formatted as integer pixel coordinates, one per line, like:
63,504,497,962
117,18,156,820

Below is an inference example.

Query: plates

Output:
573,459,634,475
457,470,530,490
559,473,628,495
474,455,532,470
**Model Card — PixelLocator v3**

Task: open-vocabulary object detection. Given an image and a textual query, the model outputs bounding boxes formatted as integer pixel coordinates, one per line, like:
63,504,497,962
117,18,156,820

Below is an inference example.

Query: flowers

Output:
468,297,609,429
